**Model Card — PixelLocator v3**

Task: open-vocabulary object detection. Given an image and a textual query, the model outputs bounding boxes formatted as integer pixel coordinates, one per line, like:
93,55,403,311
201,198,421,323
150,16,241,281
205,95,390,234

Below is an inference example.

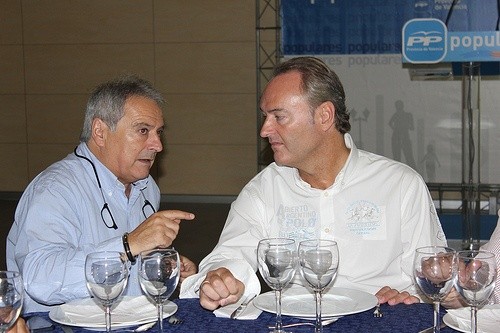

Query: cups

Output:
0,271,24,333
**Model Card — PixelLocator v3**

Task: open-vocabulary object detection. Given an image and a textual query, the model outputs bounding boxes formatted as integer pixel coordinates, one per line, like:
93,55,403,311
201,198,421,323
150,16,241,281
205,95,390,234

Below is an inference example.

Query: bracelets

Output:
122,231,138,265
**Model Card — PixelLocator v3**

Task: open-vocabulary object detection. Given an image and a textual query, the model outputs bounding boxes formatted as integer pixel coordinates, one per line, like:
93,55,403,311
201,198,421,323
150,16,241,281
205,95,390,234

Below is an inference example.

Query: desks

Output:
22,298,465,333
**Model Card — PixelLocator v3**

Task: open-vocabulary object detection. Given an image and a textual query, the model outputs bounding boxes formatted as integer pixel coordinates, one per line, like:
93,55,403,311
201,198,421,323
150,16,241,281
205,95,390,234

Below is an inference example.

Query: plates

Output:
442,306,500,333
253,286,378,317
49,295,179,331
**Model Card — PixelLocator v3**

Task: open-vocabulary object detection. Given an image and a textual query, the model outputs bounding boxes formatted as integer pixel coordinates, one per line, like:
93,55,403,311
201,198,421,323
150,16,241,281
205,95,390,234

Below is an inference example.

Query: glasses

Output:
141,190,156,219
99,187,118,230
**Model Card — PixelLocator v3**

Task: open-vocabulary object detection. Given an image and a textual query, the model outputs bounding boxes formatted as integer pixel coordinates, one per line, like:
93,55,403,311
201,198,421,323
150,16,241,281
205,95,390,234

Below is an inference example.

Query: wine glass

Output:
137,249,181,333
298,239,339,333
413,245,461,333
84,251,130,333
256,237,297,333
435,250,496,333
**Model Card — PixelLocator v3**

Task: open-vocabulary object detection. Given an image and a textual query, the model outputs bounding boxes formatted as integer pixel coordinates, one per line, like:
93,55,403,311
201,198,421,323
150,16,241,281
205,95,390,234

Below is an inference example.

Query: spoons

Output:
269,317,338,330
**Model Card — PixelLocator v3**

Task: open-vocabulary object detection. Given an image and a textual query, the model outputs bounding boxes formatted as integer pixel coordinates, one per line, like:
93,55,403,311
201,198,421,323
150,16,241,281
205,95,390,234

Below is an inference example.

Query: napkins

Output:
60,295,170,325
213,295,263,320
444,306,500,333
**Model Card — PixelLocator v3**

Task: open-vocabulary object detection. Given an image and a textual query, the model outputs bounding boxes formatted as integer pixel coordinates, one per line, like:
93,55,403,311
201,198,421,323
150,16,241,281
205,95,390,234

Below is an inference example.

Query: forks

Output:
374,303,384,318
168,313,183,326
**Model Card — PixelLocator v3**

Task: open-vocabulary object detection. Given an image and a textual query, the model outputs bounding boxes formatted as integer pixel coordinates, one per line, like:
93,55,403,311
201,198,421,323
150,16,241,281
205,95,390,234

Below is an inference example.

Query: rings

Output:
200,281,210,287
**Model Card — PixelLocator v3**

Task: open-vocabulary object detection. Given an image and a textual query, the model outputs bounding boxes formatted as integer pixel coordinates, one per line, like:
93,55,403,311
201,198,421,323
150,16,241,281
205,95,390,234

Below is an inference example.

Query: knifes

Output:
61,325,74,333
230,293,257,320
418,322,446,333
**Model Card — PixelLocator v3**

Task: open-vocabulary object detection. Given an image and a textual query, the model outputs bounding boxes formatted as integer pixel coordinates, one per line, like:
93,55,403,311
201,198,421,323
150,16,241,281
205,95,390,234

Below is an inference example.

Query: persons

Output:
435,175,500,316
194,54,447,312
0,304,31,333
7,72,196,307
